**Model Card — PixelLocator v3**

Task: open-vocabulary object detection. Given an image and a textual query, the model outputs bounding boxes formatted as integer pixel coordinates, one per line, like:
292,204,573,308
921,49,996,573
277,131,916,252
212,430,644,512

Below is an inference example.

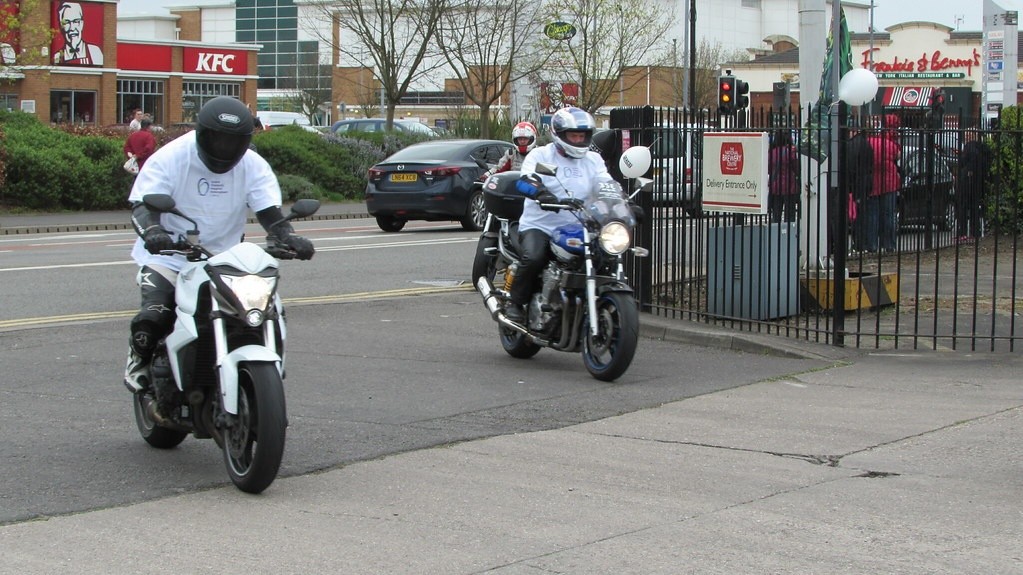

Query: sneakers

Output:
123,346,152,395
504,297,525,322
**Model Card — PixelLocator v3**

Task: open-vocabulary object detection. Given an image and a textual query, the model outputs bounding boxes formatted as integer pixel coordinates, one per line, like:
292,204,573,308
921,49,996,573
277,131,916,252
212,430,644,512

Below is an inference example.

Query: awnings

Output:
882,87,935,107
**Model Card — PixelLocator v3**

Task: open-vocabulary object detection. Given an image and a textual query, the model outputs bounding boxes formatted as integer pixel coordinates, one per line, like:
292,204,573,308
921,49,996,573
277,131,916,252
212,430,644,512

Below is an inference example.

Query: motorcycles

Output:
131,194,322,494
472,163,656,383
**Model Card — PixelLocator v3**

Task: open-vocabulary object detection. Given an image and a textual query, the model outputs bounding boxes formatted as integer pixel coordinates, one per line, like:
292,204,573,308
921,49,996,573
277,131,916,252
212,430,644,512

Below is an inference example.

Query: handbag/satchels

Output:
124,156,140,175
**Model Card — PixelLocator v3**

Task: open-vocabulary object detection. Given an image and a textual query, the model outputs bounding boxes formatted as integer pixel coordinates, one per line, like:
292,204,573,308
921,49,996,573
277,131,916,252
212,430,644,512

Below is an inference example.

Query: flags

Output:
796,5,854,163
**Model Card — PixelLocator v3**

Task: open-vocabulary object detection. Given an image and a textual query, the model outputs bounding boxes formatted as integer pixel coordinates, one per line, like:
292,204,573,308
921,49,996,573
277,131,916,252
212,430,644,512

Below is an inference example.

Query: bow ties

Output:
69,48,80,52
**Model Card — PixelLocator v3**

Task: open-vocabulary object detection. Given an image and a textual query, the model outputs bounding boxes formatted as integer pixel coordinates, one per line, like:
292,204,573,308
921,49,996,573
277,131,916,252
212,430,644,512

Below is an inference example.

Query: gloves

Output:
629,204,644,222
275,232,314,261
535,189,559,212
142,226,175,256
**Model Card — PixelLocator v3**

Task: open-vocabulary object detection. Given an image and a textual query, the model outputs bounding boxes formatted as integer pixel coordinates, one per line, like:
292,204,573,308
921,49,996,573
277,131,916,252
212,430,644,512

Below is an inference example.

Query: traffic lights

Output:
718,76,736,114
736,80,749,110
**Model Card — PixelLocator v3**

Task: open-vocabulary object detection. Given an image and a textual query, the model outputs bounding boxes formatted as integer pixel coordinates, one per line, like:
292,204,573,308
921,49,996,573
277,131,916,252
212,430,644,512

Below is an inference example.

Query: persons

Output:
129,109,143,130
123,95,315,394
768,129,799,222
847,128,902,255
502,106,643,321
479,122,540,183
124,116,157,210
952,127,981,241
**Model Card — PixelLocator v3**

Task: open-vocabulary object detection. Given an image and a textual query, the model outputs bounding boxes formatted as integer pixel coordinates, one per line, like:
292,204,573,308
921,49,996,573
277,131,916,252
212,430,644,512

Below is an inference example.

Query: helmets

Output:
195,96,254,175
551,108,596,159
512,122,537,155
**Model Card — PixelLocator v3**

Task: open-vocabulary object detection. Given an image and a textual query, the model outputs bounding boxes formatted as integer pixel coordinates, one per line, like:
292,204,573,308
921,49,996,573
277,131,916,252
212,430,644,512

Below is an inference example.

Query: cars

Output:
109,120,196,131
895,128,966,231
429,125,453,136
648,123,714,219
330,119,442,137
366,140,516,233
